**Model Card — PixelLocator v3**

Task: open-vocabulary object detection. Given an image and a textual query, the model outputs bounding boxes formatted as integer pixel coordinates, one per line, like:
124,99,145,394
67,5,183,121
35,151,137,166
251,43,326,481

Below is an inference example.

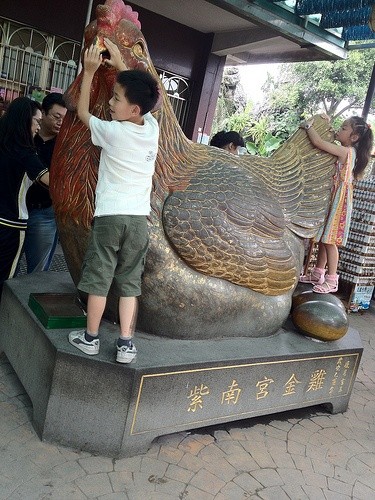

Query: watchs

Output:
304,124,313,130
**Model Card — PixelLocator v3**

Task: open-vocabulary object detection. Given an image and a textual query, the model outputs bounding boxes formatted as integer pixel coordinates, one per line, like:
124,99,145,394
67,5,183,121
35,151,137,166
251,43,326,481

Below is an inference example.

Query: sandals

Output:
298,268,326,285
312,273,339,294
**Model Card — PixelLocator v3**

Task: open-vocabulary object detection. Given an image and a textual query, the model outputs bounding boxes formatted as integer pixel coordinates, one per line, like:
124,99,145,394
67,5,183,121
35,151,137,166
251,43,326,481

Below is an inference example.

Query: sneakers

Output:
68,330,99,355
116,342,136,364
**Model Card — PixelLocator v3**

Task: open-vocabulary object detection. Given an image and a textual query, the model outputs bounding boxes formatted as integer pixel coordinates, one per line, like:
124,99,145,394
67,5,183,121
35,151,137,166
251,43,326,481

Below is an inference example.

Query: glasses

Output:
33,117,43,126
46,111,64,122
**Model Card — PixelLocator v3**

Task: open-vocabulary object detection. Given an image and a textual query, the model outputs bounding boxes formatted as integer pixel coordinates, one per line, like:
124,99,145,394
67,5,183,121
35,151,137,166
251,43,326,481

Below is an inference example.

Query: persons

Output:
299,113,375,294
0,96,49,291
69,38,161,364
209,130,247,156
22,92,67,274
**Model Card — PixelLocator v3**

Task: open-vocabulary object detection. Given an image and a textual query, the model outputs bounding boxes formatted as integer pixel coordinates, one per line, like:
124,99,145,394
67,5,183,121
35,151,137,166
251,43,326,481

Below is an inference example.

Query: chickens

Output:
45,0,341,340
